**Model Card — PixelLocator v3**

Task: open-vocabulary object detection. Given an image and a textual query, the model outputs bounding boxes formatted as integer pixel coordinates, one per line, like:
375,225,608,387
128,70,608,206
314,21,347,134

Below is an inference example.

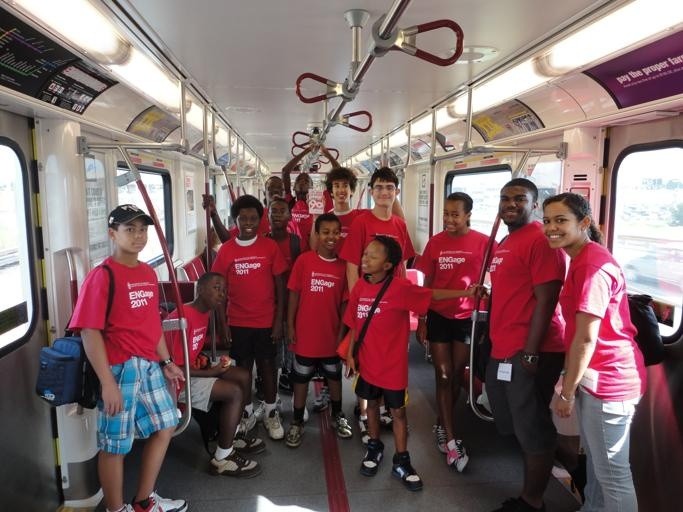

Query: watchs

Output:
519,354,540,366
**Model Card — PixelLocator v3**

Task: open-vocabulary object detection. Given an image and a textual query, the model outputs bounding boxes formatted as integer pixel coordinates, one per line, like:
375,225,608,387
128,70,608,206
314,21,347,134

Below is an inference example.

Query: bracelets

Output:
560,394,575,404
418,316,426,320
159,358,171,367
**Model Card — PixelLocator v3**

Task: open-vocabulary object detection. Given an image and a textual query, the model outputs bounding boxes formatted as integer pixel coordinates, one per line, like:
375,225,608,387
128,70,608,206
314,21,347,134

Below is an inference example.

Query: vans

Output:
618,231,681,291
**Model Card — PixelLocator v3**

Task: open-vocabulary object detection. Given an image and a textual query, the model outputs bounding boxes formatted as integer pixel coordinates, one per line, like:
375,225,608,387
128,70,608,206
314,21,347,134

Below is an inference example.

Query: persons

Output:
484,178,586,511
543,193,642,511
414,192,498,469
205,164,488,491
162,272,266,479
69,204,189,512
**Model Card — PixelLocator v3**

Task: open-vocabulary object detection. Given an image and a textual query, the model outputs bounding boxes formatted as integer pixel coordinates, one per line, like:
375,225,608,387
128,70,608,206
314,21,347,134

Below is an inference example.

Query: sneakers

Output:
379,412,393,425
392,453,423,490
449,438,469,472
122,493,188,512
331,415,353,439
359,420,371,444
360,439,383,476
278,373,308,421
492,496,546,511
434,425,449,453
209,451,262,479
234,398,284,456
310,372,330,413
286,425,304,447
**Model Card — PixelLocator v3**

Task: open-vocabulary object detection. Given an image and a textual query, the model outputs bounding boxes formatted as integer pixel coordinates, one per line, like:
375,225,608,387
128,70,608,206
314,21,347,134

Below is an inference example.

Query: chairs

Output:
182,256,206,281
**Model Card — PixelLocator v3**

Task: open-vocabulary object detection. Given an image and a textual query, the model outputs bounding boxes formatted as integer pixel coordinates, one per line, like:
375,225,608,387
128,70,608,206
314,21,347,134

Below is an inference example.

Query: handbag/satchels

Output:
36,336,100,411
628,295,665,366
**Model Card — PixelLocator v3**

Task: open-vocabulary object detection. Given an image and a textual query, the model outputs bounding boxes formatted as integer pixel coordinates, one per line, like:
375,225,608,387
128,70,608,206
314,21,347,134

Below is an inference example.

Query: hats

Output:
106,204,155,229
